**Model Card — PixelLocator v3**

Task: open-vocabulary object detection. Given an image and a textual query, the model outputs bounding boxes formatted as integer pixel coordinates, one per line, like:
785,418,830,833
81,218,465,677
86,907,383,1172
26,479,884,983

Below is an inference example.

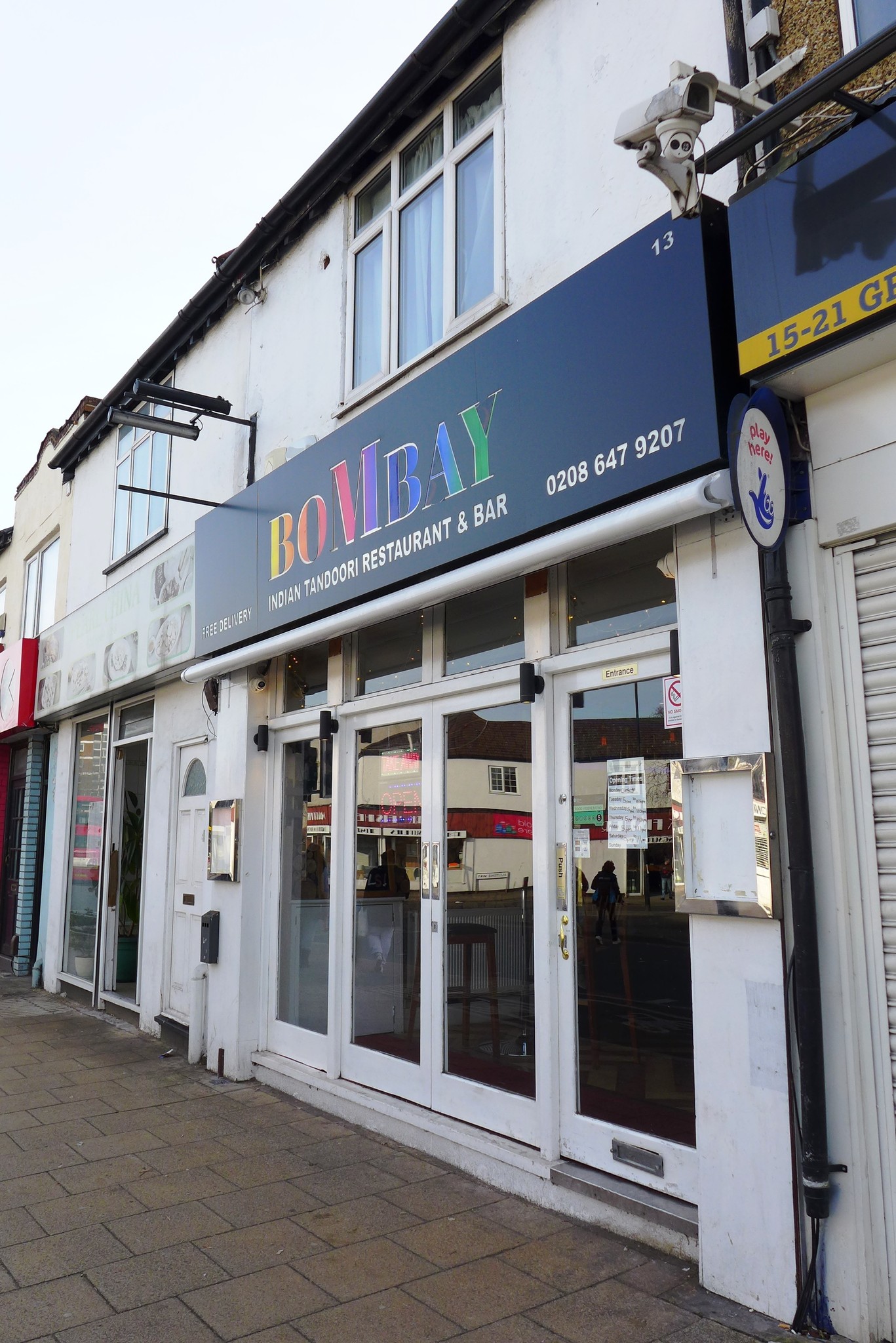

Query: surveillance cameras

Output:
250,677,267,693
615,72,719,163
656,552,675,578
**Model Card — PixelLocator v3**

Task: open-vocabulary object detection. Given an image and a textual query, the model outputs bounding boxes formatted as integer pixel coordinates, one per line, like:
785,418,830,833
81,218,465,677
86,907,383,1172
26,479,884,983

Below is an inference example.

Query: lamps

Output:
318,710,339,740
518,663,544,703
254,725,268,752
237,281,268,305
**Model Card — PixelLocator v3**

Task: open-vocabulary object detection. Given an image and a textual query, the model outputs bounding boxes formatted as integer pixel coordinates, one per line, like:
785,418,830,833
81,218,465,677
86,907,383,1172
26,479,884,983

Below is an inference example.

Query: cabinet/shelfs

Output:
294,904,394,1037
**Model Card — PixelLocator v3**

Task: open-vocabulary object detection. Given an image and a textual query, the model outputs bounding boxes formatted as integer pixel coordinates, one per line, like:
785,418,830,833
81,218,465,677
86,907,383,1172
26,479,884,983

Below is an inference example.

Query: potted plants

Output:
101,788,144,982
66,907,99,979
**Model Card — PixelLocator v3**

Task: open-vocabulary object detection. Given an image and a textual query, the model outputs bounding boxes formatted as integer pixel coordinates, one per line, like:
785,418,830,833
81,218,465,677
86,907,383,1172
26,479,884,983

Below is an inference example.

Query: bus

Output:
72,795,103,884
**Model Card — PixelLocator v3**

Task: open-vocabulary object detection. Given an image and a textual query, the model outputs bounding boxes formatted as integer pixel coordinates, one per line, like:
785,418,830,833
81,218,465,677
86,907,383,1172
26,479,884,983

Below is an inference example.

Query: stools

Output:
406,923,500,1053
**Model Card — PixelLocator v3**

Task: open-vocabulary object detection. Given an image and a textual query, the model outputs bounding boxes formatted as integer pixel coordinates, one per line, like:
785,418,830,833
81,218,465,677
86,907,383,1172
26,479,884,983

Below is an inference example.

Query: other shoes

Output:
595,936,603,945
612,938,620,945
660,898,664,900
669,896,674,899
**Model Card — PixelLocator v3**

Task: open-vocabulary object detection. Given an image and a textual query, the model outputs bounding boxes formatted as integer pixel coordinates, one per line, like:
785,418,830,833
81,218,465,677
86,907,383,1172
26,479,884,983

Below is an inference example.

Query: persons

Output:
576,865,589,936
362,849,410,973
590,861,624,944
299,843,329,967
659,858,674,900
